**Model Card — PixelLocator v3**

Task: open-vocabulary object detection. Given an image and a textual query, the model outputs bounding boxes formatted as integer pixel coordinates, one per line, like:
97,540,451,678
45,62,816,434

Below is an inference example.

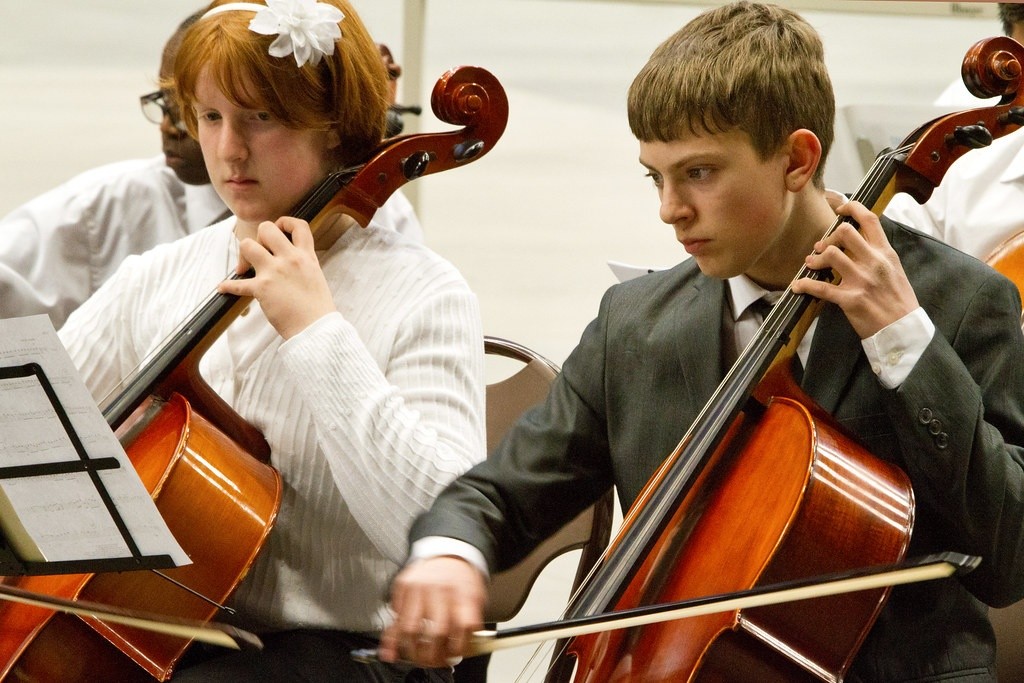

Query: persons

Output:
376,1,1024,683
0,0,487,683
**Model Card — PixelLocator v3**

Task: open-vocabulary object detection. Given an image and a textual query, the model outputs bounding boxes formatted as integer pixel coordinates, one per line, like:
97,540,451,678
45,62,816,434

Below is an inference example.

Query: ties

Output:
750,297,805,386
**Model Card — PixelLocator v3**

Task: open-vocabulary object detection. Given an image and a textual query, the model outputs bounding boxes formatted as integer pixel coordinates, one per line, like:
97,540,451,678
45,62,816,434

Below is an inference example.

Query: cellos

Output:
0,66,509,683
497,33,1024,682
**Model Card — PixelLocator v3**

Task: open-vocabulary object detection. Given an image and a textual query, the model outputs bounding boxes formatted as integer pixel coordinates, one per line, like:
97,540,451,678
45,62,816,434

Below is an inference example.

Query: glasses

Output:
140,91,189,133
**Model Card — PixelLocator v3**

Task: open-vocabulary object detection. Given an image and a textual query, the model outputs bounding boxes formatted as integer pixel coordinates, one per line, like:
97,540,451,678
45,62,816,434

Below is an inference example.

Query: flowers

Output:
249,0,347,68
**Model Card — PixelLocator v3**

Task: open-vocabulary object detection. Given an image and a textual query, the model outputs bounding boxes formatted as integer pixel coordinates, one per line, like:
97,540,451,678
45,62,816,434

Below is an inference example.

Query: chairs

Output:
451,332,616,683
